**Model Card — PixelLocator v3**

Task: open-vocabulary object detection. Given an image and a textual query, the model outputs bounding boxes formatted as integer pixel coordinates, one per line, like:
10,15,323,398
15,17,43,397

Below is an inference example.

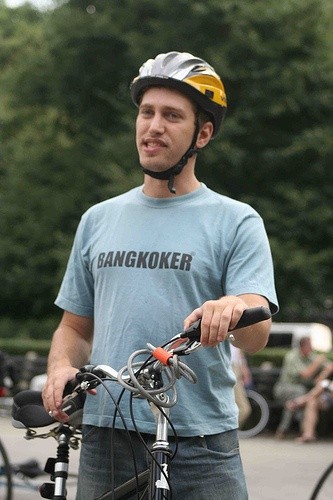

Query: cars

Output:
0,350,83,481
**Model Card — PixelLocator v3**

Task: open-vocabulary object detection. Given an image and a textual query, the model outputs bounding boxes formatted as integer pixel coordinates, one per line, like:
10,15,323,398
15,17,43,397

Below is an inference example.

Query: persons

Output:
228,340,252,428
37,60,280,500
272,337,333,442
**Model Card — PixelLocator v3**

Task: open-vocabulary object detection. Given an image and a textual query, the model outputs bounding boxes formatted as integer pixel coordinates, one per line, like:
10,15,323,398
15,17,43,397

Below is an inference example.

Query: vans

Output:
238,321,333,434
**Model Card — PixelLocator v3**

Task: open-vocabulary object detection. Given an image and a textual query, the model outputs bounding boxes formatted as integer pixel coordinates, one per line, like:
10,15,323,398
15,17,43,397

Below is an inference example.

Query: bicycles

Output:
0,300,270,500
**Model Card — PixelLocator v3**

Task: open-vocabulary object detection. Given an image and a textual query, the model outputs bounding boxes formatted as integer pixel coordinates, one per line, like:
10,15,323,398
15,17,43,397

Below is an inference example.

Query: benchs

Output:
244,365,333,435
0,355,48,411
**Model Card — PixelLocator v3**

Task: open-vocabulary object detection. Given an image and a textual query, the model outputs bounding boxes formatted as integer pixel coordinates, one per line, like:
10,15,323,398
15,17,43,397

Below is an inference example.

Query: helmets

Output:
129,51,226,139
